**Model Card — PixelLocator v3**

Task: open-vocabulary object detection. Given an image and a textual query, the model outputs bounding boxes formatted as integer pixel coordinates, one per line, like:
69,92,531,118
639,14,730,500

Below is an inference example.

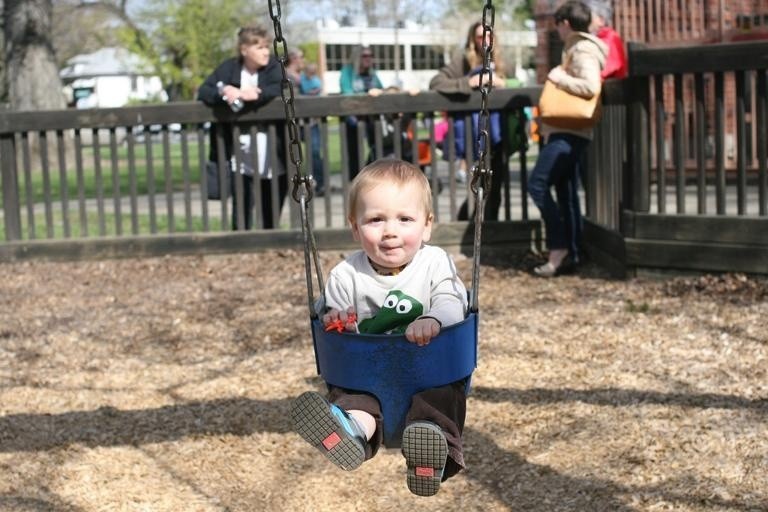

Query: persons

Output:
524,2,606,278
198,24,291,233
280,44,466,196
585,3,628,81
426,22,509,222
289,156,469,497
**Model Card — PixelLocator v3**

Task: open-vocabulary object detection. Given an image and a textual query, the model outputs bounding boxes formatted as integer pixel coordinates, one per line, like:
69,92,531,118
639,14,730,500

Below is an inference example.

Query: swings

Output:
266,1,497,449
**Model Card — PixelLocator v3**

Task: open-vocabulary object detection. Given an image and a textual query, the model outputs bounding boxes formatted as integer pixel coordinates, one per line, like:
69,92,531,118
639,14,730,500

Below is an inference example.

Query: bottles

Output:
216,80,244,113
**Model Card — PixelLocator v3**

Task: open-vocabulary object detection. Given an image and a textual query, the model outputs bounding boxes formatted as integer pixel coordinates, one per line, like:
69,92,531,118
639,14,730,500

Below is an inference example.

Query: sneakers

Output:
402,419,449,496
290,390,371,470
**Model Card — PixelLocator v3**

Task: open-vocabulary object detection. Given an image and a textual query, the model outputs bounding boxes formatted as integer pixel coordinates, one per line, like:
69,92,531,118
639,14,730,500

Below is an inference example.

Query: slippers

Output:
535,258,573,277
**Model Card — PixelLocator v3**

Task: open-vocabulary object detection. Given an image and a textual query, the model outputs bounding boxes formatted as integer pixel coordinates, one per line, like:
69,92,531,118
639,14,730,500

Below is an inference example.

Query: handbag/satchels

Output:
208,158,231,198
538,74,602,128
